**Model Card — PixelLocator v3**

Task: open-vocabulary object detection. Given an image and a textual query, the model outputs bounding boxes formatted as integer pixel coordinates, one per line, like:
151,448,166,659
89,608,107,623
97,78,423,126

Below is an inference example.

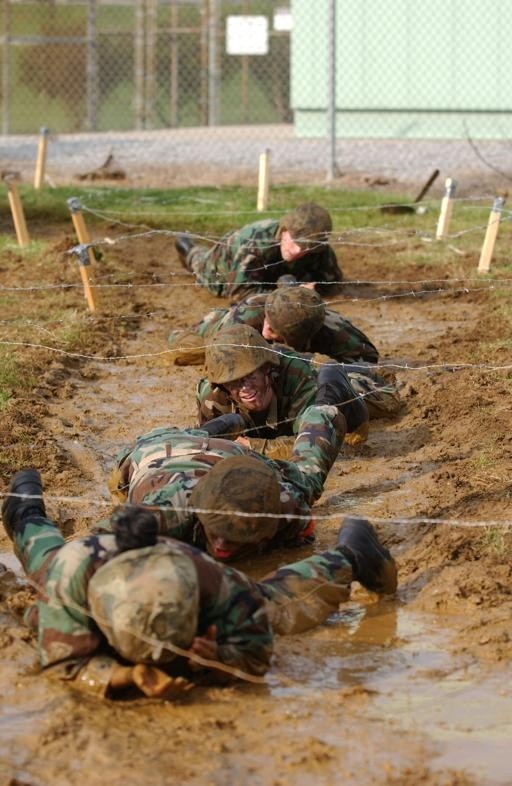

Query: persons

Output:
194,324,402,439
197,273,380,366
175,202,344,304
1,466,399,700
87,361,369,566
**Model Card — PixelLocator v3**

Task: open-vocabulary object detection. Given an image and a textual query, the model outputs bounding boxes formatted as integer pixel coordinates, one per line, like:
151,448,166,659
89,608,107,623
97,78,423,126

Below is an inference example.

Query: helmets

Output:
86,542,201,666
189,456,281,546
264,286,325,352
275,203,332,254
205,324,281,388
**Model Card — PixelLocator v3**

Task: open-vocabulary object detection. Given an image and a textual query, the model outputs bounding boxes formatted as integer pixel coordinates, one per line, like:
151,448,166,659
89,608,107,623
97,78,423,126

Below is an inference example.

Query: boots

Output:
276,273,299,287
174,237,194,269
199,413,246,441
1,469,46,542
315,359,369,433
334,514,397,595
